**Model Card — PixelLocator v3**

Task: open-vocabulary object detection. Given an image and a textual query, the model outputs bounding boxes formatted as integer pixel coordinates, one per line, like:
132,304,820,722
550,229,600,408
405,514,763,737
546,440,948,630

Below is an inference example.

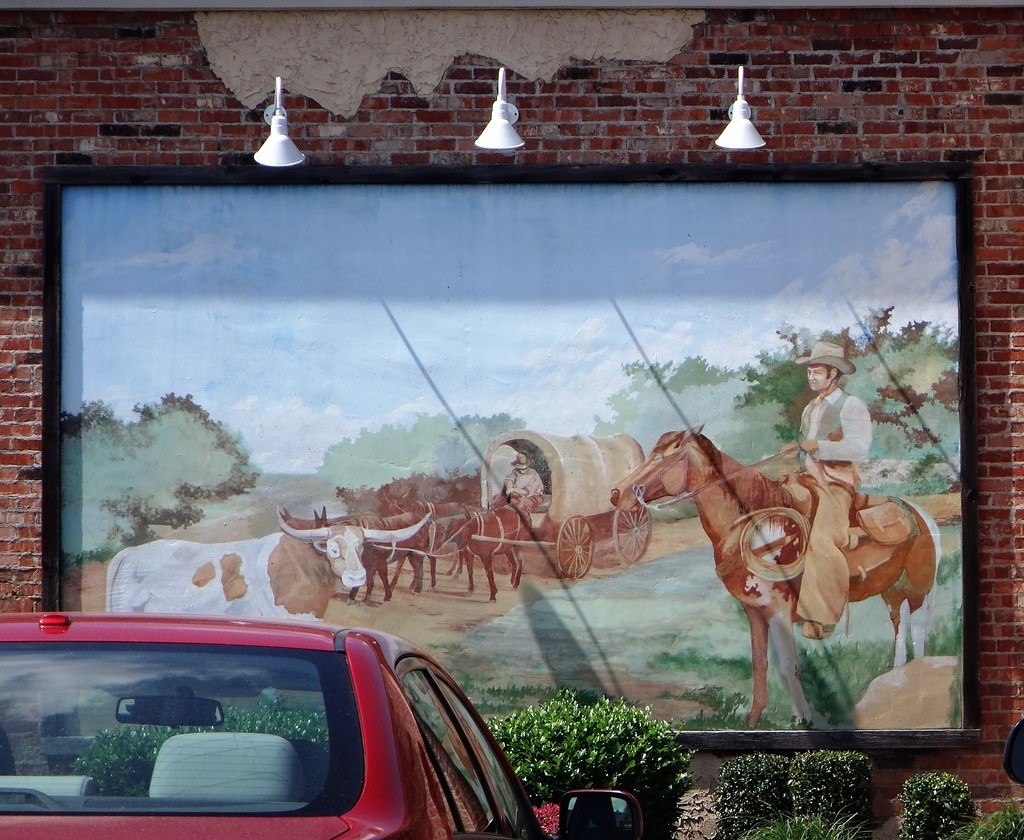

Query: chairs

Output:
149,731,307,806
0,775,94,796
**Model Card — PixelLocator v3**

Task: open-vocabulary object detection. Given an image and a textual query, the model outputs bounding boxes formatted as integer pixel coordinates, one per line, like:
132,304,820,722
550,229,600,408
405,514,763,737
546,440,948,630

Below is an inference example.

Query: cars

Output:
0,610,644,839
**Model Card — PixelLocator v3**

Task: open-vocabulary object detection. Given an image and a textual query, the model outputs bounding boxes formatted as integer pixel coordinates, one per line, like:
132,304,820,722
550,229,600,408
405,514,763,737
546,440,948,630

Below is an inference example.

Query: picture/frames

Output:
41,161,981,754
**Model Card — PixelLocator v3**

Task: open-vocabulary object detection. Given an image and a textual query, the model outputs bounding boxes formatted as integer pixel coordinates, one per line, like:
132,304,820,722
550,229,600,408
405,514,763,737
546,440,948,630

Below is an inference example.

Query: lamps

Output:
715,66,766,149
254,76,305,166
474,67,525,150
